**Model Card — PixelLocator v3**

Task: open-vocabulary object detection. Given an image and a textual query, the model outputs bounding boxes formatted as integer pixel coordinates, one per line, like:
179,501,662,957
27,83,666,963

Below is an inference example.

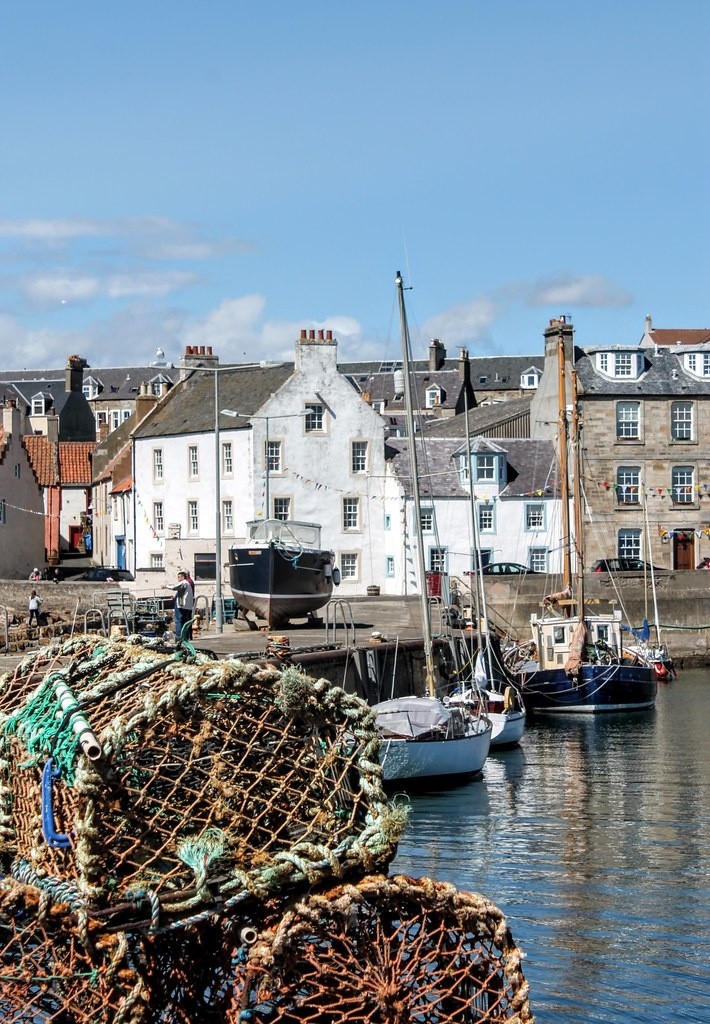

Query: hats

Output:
34,568,38,572
179,570,190,574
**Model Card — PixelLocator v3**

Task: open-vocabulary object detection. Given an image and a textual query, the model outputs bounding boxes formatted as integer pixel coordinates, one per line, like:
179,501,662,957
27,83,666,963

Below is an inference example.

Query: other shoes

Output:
27,625,32,629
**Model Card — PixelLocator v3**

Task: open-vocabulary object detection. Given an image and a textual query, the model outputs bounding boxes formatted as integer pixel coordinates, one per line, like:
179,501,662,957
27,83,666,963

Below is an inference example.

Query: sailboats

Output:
624,481,674,680
378,269,492,792
505,323,658,714
443,387,527,744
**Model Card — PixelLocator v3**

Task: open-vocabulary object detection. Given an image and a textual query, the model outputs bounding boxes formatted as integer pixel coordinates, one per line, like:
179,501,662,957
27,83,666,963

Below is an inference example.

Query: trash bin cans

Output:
425,571,448,599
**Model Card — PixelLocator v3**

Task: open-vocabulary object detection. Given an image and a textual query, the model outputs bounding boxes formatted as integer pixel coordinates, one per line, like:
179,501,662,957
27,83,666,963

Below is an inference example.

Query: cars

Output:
590,558,669,572
463,562,546,575
64,566,134,581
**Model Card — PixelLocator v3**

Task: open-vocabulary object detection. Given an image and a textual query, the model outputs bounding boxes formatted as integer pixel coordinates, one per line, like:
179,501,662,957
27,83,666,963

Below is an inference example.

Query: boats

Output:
228,517,341,630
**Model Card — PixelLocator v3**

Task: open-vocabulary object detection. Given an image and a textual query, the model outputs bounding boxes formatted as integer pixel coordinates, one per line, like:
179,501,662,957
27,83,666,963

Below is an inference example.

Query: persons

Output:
162,568,195,641
28,590,42,628
696,557,710,569
29,567,52,581
51,568,64,581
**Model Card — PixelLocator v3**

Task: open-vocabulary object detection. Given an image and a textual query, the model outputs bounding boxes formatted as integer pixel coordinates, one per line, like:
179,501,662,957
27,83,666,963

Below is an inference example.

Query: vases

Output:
619,437,638,441
675,437,689,441
673,502,694,505
619,502,640,506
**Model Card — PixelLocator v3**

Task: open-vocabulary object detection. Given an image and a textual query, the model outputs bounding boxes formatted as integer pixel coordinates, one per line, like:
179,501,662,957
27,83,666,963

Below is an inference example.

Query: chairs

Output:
625,562,628,569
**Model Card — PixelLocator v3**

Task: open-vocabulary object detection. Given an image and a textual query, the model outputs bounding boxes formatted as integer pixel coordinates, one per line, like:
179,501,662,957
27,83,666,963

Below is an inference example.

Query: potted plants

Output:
48,555,59,565
367,585,380,596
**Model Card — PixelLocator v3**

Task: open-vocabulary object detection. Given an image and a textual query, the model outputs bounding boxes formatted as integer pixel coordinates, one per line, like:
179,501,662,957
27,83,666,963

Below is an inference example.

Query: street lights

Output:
220,405,315,521
148,357,284,633
